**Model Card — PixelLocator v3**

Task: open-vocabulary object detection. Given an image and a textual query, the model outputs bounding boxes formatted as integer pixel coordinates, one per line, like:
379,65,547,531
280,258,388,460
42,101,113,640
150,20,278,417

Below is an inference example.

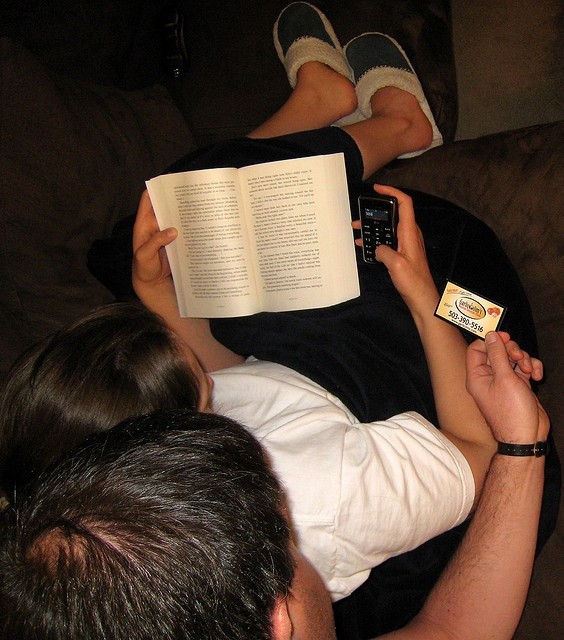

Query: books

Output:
142,151,363,322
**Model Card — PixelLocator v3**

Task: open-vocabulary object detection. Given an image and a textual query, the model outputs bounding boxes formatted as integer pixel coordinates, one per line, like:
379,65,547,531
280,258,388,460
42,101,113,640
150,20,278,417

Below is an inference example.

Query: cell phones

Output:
358,193,399,265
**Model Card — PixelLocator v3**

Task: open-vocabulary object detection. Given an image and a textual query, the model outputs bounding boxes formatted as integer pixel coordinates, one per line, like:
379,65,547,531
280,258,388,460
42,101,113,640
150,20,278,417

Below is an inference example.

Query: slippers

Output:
272,1,354,118
341,30,445,161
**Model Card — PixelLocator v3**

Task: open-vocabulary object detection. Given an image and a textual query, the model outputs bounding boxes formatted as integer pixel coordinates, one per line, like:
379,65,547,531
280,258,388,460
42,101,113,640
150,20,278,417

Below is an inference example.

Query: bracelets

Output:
495,440,551,457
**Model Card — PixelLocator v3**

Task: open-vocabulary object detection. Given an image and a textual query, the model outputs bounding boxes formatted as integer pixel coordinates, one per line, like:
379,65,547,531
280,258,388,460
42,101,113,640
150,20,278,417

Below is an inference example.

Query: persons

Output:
1,330,550,640
